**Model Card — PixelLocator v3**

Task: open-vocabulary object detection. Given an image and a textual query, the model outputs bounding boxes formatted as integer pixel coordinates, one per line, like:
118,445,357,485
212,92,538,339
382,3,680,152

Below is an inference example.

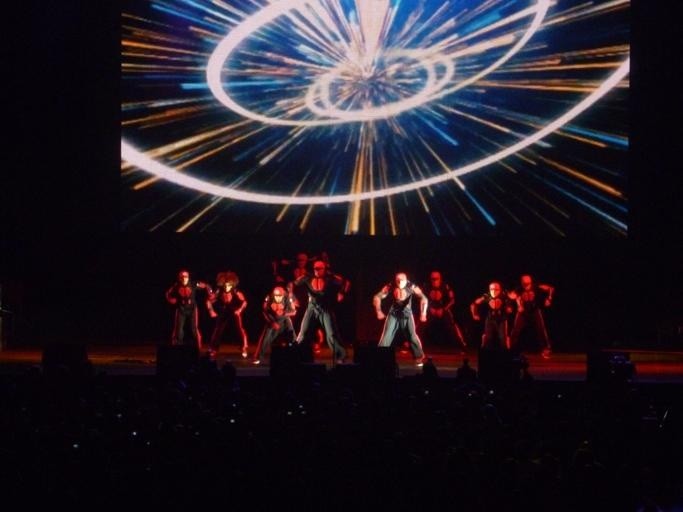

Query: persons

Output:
165,251,556,370
2,380,682,510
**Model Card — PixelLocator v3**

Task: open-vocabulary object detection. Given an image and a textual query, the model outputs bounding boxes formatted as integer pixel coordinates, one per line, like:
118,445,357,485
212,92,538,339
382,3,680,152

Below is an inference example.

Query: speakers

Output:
41,342,89,369
357,343,396,377
586,349,631,381
477,339,528,380
156,343,193,375
270,341,308,377
